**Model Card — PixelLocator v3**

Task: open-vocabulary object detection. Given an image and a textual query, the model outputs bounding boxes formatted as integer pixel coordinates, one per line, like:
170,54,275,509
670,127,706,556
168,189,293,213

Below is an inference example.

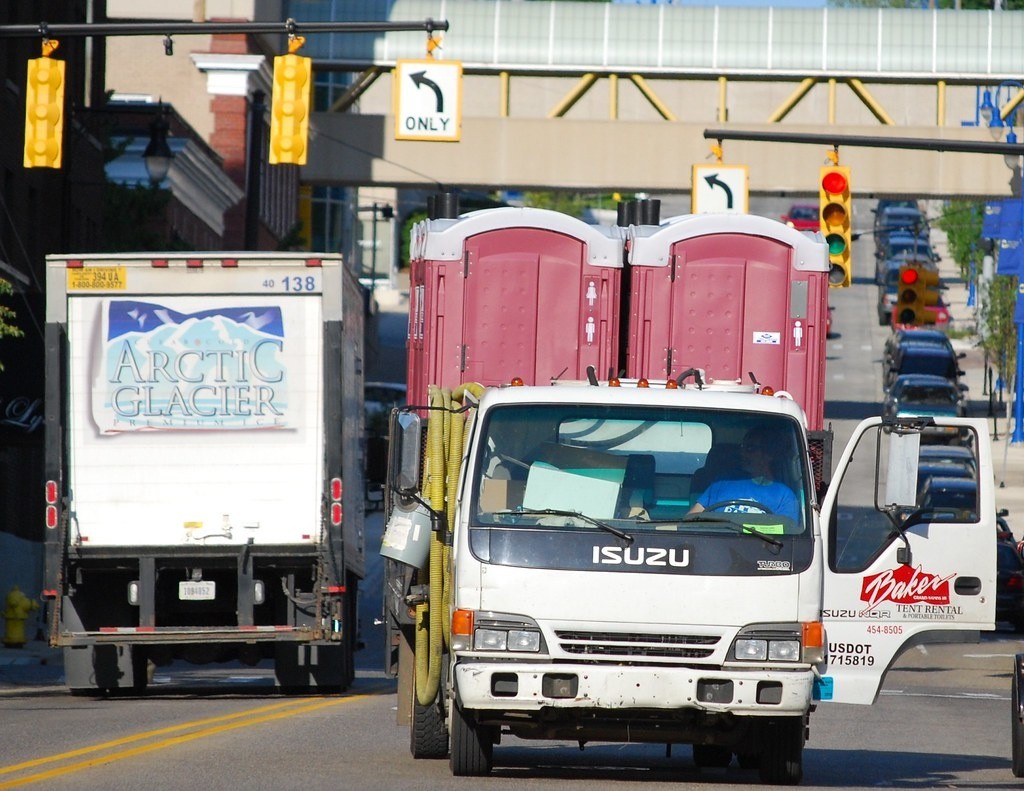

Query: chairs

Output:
691,443,744,511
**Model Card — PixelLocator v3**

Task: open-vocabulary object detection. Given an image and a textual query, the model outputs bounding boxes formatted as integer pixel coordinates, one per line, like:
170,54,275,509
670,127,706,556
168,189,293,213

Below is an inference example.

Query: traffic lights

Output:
893,267,921,327
821,164,856,290
918,265,940,328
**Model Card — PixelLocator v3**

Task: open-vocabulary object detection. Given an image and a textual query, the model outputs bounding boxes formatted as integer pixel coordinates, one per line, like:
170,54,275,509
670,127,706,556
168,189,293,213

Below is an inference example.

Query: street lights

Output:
76,97,170,251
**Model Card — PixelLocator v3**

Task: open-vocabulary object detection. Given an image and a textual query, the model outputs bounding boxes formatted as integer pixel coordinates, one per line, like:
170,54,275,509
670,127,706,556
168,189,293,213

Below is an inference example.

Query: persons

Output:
685,425,800,524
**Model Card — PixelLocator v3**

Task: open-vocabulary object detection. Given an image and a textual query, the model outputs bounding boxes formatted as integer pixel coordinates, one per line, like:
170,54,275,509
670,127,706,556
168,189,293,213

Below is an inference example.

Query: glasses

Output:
739,442,768,452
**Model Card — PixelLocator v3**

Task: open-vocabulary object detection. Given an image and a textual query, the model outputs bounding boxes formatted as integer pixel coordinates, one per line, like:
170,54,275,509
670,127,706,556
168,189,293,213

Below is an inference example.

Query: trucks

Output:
44,252,362,693
379,366,1000,791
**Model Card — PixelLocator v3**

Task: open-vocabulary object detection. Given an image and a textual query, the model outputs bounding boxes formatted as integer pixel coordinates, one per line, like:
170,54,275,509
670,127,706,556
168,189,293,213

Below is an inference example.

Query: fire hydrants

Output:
0,584,40,649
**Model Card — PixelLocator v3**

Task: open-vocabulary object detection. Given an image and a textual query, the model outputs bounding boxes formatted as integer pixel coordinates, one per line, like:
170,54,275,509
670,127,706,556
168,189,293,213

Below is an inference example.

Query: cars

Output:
891,297,951,333
871,198,950,325
912,429,1024,632
883,331,969,417
780,204,821,233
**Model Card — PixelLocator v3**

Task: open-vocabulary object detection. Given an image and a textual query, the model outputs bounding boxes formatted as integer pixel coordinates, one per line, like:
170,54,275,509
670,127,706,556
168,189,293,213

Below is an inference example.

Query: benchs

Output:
494,455,656,510
